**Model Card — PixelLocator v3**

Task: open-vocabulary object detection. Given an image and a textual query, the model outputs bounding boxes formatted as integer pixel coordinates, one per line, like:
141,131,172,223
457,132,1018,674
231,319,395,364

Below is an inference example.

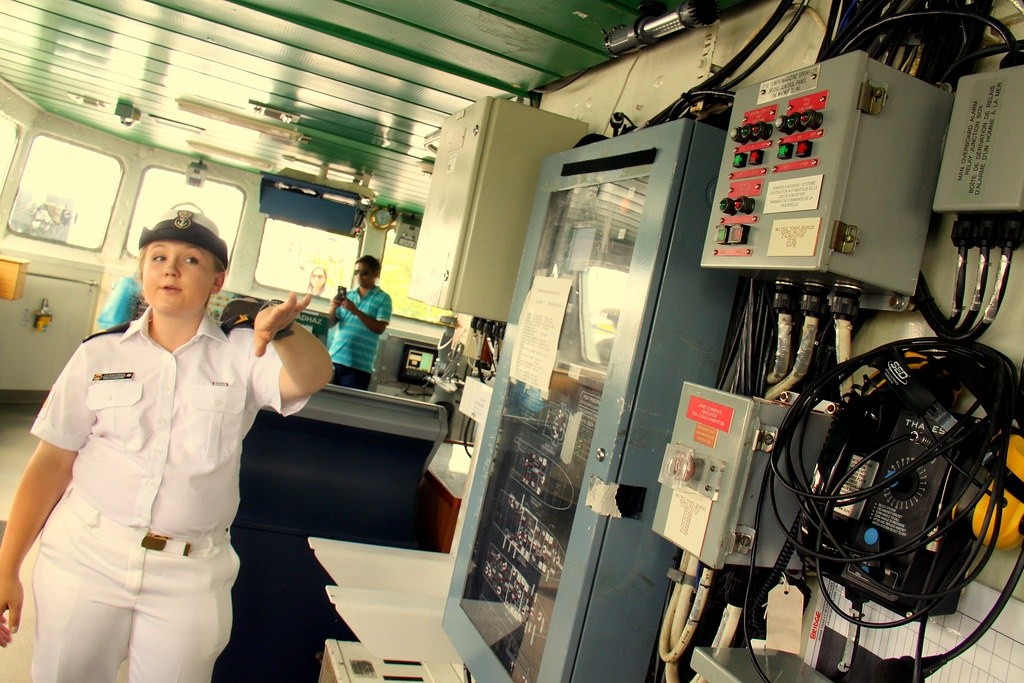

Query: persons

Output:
307,266,329,298
329,255,392,390
0,209,333,683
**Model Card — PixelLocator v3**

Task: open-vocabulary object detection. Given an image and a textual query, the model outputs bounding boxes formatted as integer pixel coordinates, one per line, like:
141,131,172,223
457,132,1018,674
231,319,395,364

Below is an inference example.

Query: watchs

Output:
258,299,294,339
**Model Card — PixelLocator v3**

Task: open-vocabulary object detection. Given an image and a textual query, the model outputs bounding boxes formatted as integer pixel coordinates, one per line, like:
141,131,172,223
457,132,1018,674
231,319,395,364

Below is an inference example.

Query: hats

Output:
139,201,228,271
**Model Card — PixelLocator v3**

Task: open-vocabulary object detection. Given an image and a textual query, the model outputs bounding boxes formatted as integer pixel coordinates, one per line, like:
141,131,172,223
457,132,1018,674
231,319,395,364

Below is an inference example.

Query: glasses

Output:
353,270,372,276
311,274,324,281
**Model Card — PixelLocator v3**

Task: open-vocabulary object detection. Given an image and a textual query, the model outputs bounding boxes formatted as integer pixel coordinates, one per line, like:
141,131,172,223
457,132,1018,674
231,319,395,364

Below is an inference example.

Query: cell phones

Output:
338,285,346,300
